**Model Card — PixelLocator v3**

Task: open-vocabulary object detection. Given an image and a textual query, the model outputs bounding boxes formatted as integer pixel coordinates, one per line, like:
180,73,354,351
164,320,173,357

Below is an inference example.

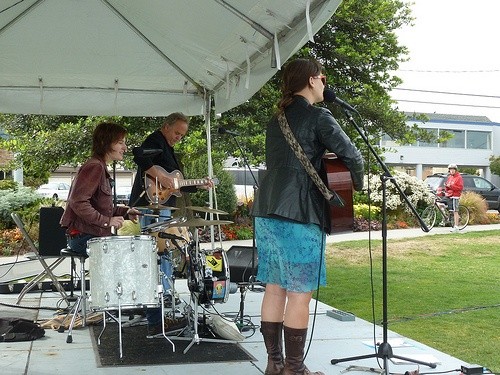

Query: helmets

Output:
448,163,457,169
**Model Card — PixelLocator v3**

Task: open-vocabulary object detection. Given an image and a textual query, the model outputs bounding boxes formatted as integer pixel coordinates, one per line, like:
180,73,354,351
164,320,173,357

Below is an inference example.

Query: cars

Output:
34,181,71,201
113,186,132,205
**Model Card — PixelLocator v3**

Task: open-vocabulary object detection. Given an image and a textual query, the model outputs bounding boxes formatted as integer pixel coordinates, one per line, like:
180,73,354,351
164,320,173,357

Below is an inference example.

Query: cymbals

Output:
165,217,234,227
141,204,180,210
184,205,230,215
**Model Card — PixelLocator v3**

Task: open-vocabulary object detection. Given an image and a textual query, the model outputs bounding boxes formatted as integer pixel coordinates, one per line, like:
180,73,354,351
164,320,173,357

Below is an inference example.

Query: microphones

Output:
218,128,239,136
158,232,184,240
323,89,359,115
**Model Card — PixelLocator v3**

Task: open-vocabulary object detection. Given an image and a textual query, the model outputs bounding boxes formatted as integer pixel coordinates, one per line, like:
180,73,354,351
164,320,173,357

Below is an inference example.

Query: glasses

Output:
312,75,326,85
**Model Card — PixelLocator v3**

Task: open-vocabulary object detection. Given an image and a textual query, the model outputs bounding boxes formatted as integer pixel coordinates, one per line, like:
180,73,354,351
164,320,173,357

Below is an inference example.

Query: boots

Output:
260,321,284,375
284,325,324,375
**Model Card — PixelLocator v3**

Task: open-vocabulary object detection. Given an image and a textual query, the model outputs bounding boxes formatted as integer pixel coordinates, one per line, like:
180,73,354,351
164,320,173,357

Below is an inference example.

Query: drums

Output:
188,247,230,304
142,216,192,255
86,234,160,312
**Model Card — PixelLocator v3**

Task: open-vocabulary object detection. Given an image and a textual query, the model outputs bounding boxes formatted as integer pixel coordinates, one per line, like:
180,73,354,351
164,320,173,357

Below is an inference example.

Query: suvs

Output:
418,172,500,212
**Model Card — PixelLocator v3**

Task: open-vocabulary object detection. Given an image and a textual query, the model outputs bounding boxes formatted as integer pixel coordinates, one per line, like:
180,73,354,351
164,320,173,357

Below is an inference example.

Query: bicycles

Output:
419,190,469,233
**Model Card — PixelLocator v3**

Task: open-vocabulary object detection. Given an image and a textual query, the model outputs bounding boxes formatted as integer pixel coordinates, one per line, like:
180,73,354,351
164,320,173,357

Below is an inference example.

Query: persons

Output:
128,111,213,308
248,59,364,375
59,122,191,339
437,163,464,232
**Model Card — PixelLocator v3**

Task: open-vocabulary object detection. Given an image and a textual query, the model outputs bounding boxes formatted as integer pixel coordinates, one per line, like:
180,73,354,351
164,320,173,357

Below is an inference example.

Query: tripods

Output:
160,291,237,354
330,106,436,375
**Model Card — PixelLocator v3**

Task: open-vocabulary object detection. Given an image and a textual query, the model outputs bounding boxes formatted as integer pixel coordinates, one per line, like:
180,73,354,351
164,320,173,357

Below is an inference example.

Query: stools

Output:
59,249,125,344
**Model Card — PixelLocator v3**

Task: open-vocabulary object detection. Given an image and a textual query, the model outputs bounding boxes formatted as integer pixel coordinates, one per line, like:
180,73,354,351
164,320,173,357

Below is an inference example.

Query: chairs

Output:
10,212,70,305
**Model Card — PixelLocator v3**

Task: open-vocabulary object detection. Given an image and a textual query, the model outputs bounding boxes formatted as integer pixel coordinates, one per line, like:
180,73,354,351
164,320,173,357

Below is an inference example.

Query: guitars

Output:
140,165,220,205
317,152,356,235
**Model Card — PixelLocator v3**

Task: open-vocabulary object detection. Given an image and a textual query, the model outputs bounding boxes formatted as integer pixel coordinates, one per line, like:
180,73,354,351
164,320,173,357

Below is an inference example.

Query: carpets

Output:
89,321,258,368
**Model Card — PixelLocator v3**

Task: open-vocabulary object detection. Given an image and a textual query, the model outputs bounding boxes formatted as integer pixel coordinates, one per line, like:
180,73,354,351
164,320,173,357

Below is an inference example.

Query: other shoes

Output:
441,216,449,223
159,294,181,306
148,318,189,336
450,225,460,232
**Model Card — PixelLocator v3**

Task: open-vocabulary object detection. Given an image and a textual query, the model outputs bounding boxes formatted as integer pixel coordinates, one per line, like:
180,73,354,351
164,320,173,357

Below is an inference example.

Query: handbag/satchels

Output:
0,317,45,343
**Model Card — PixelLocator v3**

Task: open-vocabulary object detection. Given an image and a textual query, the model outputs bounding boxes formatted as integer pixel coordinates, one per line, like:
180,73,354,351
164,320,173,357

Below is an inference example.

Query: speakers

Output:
213,246,261,282
39,206,67,256
